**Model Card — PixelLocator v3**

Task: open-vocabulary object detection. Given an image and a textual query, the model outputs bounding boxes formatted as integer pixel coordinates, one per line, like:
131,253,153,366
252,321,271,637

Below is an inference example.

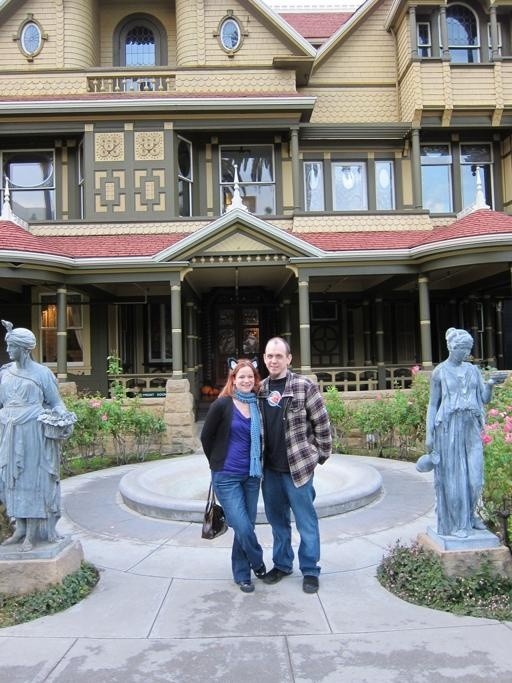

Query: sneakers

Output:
236,580,254,592
255,564,266,578
261,568,287,584
302,574,319,595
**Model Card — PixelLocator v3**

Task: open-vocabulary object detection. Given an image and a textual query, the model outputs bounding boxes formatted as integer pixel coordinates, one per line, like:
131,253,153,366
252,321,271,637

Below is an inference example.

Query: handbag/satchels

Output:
200,480,226,539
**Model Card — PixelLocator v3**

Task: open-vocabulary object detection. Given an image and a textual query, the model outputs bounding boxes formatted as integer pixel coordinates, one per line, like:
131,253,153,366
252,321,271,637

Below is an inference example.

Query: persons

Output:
0,320,78,552
414,328,507,539
200,359,267,593
256,338,331,593
